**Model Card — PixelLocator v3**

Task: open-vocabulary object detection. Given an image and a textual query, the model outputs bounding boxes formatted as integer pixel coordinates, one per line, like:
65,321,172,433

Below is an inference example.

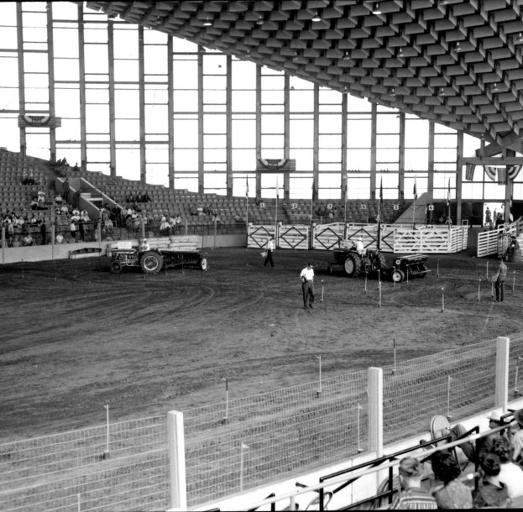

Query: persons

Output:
311,197,505,232
298,261,317,311
354,237,364,254
1,156,264,256
502,236,519,258
384,406,523,511
262,237,276,266
491,256,509,303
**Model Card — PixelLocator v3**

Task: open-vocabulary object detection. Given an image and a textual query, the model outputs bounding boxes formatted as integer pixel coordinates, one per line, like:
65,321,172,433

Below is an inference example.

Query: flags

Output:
497,167,507,185
465,162,476,181
